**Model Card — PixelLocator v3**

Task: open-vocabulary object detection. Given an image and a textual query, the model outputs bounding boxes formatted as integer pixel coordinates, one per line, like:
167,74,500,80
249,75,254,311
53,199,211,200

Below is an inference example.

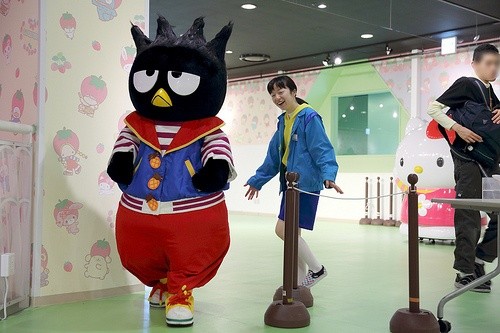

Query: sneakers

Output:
454,273,492,292
300,264,328,288
474,262,492,286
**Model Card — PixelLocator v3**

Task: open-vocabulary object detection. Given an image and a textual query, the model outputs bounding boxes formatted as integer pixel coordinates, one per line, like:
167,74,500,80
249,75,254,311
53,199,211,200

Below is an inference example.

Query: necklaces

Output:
475,79,492,111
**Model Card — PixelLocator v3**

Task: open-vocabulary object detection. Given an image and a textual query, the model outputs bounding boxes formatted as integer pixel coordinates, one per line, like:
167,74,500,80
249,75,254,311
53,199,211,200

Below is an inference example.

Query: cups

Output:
482,177,500,201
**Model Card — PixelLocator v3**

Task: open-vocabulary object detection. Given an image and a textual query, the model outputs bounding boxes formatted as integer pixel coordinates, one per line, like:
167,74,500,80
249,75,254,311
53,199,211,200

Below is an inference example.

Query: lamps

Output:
321,52,331,67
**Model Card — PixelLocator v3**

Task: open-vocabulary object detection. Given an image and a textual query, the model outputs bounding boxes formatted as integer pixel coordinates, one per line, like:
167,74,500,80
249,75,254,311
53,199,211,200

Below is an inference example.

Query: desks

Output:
430,198,500,333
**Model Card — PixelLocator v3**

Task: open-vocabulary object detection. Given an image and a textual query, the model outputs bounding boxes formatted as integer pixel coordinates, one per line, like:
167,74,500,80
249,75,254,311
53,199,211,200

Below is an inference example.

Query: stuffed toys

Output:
108,13,237,327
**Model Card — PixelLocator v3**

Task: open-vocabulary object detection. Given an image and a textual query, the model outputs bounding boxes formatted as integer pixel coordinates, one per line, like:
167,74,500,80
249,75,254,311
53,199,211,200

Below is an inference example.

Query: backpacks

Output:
438,99,500,178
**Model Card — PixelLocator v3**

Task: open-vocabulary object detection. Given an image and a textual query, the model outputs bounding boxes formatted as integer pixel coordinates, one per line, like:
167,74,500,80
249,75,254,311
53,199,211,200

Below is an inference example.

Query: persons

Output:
244,76,342,287
427,44,500,292
